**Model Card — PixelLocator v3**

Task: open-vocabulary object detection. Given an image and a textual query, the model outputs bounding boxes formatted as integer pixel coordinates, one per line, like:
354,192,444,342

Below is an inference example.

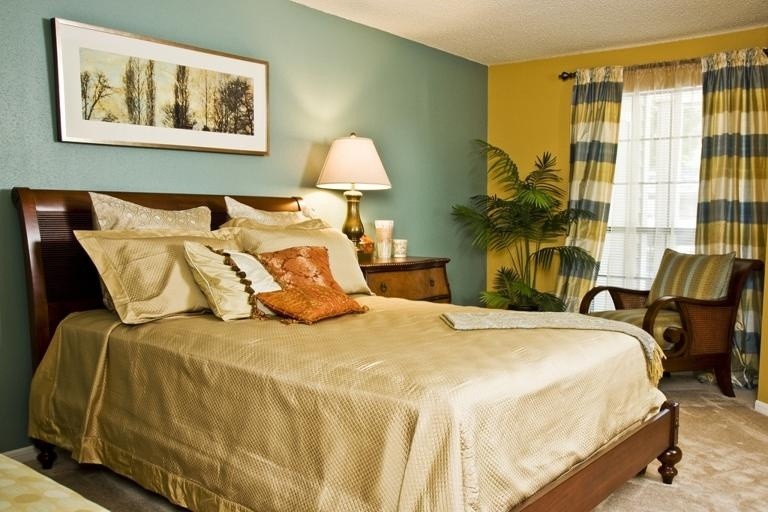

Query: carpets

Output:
0,453,112,512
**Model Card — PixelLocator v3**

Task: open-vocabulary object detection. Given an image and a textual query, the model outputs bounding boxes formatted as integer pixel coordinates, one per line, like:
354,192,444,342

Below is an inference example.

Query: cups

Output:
391,237,408,258
374,218,394,259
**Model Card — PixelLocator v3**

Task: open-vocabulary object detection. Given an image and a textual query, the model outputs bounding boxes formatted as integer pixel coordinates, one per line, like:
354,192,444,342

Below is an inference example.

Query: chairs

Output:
577,250,761,399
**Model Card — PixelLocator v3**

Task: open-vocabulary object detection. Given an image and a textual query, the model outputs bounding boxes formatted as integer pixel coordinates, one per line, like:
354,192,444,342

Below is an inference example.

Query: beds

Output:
12,185,681,512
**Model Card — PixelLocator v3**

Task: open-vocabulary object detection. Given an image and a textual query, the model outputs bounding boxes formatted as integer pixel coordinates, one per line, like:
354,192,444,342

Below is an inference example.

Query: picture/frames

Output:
52,17,270,156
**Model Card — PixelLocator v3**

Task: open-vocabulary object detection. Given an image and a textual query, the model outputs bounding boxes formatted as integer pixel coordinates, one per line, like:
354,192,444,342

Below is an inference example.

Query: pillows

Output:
644,248,735,311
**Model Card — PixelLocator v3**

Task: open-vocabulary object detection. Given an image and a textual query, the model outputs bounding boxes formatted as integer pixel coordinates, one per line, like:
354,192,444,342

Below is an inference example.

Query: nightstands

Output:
358,256,452,305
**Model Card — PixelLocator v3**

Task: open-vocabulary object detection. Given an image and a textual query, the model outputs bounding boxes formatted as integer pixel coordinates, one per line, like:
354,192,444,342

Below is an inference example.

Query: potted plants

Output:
448,137,603,312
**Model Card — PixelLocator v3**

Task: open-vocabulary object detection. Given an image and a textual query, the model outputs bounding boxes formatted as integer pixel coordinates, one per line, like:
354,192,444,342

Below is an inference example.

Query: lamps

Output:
315,132,393,252
